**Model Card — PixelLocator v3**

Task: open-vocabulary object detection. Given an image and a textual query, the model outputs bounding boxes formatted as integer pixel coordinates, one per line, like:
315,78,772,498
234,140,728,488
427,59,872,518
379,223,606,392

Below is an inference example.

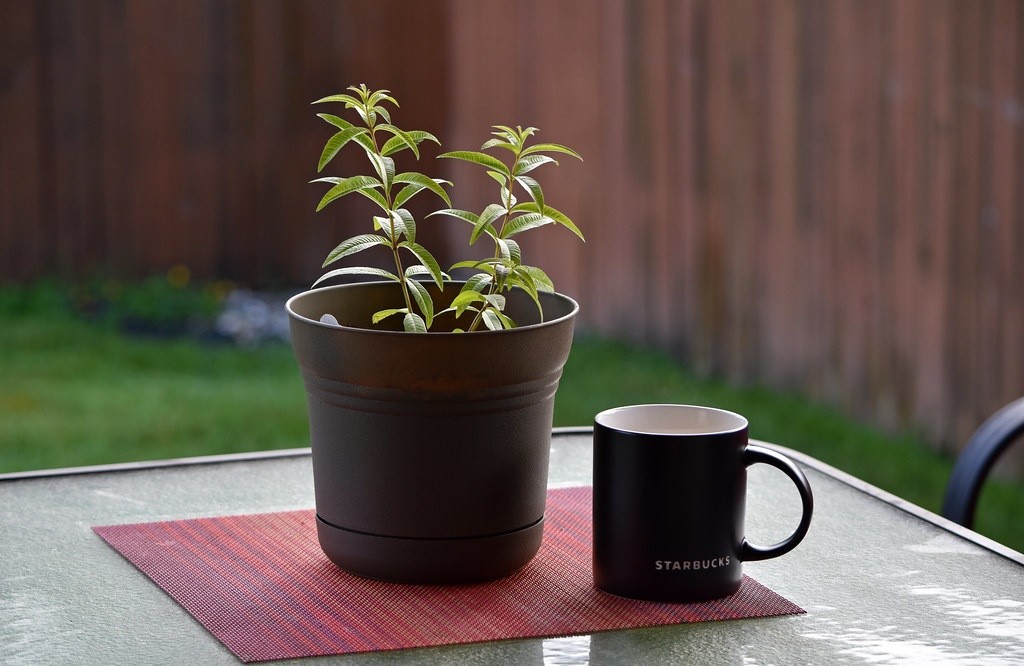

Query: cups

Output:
592,403,814,602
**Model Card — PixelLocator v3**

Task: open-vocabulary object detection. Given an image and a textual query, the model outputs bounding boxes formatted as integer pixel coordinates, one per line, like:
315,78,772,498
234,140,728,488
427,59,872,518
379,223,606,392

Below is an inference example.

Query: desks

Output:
0,427,1024,666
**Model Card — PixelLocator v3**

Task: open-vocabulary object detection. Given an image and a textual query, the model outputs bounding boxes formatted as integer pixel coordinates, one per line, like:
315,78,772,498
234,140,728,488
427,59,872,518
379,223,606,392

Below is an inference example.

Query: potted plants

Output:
286,82,587,590
